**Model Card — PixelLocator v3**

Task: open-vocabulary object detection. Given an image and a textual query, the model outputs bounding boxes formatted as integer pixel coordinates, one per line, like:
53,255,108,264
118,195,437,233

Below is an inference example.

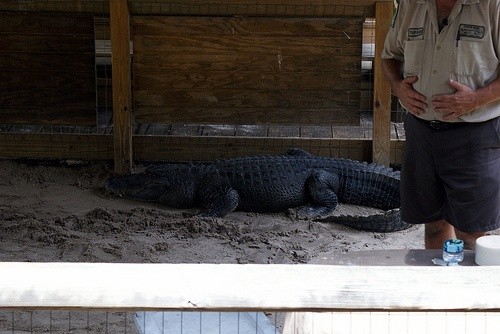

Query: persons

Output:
381,0,500,250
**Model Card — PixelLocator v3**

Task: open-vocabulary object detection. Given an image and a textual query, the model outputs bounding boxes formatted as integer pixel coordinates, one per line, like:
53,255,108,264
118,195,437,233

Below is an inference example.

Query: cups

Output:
442,239,465,263
